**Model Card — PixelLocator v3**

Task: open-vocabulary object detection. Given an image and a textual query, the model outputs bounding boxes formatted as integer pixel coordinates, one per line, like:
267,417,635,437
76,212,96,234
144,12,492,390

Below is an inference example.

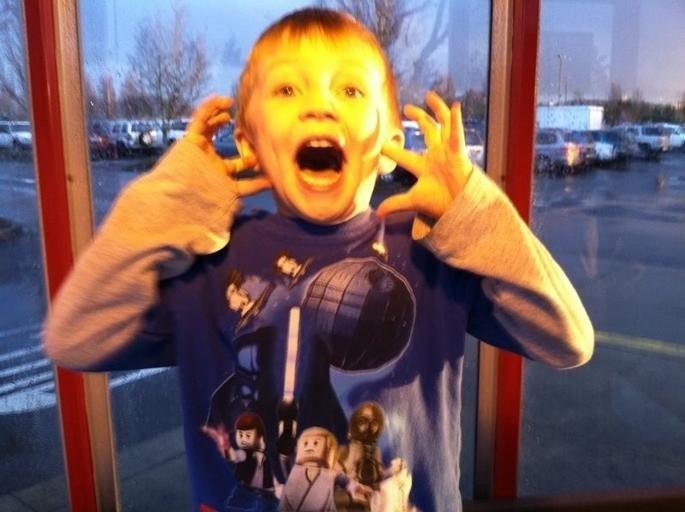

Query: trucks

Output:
527,104,608,133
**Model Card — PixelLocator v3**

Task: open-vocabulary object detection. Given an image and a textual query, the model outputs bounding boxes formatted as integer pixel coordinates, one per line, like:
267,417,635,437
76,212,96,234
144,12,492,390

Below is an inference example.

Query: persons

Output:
41,8,595,510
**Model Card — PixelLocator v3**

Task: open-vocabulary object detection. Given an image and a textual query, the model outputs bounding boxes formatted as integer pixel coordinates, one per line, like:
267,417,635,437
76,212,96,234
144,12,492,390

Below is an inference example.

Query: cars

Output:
394,119,486,176
570,129,630,167
532,127,597,176
88,116,240,161
610,120,684,159
1,120,33,160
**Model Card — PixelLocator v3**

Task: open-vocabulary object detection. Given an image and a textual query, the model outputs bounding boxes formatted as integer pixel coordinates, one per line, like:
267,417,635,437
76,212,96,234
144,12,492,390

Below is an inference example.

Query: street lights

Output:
557,50,570,107
